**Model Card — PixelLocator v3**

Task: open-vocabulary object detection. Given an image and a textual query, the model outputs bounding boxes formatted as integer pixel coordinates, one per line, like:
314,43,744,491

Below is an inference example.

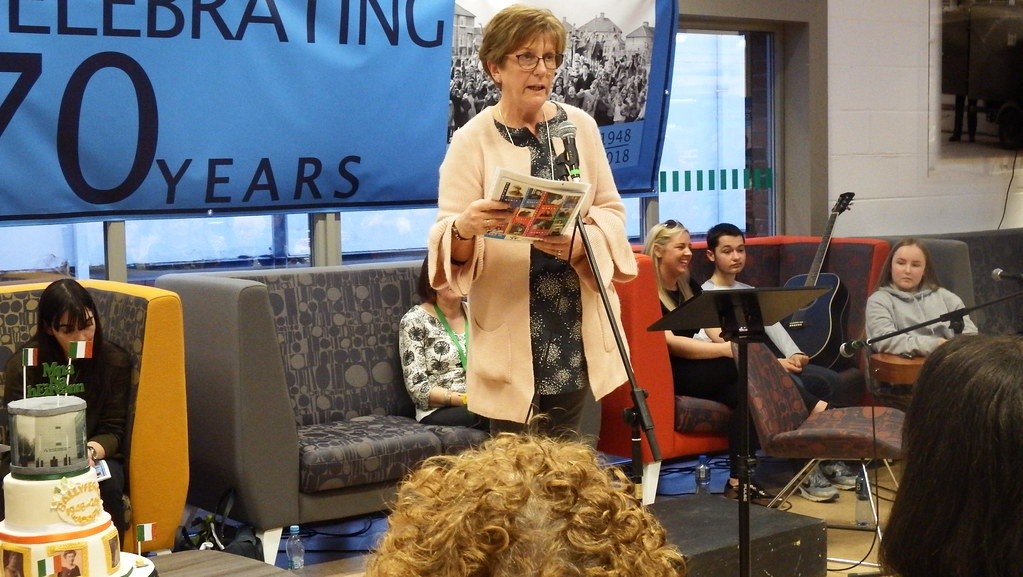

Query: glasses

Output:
665,220,683,228
506,53,563,70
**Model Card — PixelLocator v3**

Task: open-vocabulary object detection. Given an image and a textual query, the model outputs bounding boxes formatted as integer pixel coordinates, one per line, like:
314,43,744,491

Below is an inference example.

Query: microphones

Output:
557,121,581,184
992,269,1023,281
840,340,865,357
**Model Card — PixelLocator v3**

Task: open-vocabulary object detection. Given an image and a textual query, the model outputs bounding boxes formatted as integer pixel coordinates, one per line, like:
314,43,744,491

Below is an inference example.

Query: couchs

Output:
153,261,489,570
0,278,189,566
595,232,891,484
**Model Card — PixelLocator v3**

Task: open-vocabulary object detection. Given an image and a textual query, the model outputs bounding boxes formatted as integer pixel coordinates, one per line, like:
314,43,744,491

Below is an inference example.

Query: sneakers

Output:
821,461,858,490
793,465,839,502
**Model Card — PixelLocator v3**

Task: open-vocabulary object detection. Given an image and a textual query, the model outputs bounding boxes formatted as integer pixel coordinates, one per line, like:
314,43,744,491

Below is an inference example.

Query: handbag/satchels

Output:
171,487,265,563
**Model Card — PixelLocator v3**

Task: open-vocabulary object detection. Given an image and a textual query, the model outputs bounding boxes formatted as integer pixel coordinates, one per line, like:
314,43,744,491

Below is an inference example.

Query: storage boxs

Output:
643,491,827,577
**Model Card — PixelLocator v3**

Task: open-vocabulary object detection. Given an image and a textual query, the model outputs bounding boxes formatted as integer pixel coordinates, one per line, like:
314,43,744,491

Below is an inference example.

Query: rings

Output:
557,249,561,258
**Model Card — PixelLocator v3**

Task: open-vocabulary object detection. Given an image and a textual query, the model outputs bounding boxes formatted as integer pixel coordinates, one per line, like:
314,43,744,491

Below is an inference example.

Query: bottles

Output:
695,456,711,497
855,469,872,526
286,526,305,571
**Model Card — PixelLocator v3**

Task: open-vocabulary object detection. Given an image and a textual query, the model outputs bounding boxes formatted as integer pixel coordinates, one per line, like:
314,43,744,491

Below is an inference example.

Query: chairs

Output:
733,337,904,569
912,239,972,318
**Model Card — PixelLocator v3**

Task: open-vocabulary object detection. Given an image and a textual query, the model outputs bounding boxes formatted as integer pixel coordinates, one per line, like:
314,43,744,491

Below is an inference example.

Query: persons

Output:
363,411,688,577
4,279,133,551
489,185,579,238
865,238,978,358
876,333,1023,577
642,219,784,508
448,44,650,144
398,254,494,436
699,222,869,503
427,4,640,452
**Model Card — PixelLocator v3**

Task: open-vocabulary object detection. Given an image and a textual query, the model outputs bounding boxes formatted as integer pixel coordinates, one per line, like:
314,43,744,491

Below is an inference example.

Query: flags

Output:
22,348,38,366
68,341,92,359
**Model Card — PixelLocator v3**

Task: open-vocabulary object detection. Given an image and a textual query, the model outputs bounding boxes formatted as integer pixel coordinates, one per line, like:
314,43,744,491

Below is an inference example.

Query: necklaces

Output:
497,103,555,181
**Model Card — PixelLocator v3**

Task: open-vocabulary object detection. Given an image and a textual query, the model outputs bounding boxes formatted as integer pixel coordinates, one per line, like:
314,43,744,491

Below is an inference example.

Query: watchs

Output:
452,222,475,241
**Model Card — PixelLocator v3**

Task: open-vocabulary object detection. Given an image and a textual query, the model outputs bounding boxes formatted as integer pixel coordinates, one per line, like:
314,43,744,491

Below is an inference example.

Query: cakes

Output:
0,340,121,577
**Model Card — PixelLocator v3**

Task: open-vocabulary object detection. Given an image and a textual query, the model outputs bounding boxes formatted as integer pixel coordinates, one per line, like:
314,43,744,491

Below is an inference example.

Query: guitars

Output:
778,192,855,371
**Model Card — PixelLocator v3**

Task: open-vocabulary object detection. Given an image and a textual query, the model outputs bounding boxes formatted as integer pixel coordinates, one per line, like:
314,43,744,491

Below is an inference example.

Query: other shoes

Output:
724,477,782,507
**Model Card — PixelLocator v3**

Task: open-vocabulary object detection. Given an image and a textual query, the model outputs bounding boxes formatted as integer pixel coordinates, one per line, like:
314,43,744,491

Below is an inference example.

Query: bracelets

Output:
446,390,452,404
